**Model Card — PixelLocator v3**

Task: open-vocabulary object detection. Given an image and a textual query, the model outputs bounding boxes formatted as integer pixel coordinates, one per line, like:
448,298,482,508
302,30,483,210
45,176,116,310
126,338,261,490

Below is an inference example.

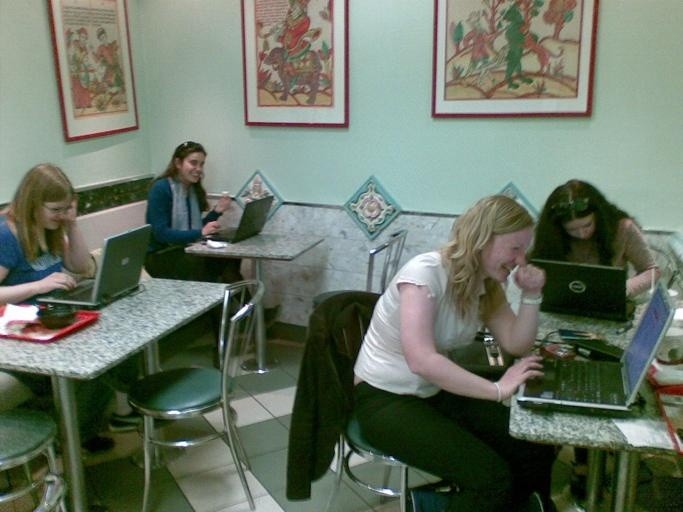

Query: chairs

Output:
649,242,679,291
315,292,458,512
0,403,70,512
311,231,409,314
126,280,266,512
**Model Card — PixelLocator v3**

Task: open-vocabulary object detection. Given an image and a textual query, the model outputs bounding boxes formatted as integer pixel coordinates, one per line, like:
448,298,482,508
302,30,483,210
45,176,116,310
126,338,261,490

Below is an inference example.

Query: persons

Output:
459,9,493,89
528,179,662,503
0,162,121,452
144,141,282,369
66,27,123,110
277,0,316,61
351,195,545,511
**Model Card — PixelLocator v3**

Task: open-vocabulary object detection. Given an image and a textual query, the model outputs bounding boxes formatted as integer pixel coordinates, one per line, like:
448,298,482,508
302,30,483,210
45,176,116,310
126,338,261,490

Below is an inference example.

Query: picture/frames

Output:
431,1,601,118
48,1,140,143
241,1,351,129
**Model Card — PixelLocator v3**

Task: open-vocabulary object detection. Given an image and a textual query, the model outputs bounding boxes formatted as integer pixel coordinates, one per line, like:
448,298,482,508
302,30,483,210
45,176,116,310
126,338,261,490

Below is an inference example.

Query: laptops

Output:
518,277,677,418
206,195,275,243
37,223,154,309
526,259,636,322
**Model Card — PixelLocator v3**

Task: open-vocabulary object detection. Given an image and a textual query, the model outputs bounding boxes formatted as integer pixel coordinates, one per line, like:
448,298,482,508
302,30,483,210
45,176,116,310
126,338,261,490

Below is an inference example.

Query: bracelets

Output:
520,296,544,304
494,382,503,403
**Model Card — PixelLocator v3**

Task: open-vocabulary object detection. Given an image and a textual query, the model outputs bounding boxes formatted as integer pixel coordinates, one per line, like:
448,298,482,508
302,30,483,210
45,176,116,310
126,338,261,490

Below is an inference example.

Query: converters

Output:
577,340,624,364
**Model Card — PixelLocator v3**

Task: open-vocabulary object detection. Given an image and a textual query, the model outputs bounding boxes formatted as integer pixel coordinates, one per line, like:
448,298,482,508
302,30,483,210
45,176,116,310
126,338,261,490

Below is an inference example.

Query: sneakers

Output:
108,409,157,432
264,305,283,329
409,489,422,512
570,470,598,501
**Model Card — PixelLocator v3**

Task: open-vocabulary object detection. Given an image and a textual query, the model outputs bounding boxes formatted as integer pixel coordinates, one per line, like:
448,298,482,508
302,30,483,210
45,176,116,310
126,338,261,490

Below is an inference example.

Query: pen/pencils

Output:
616,324,633,334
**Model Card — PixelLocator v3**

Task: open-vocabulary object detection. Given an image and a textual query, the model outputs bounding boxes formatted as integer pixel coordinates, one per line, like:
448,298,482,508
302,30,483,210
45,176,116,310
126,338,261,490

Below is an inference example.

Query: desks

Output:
508,312,683,512
0,283,239,511
185,231,325,376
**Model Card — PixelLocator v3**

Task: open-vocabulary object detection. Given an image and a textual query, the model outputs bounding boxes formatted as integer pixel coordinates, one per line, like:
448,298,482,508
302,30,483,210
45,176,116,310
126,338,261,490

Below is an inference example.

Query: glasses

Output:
551,196,593,217
43,205,72,213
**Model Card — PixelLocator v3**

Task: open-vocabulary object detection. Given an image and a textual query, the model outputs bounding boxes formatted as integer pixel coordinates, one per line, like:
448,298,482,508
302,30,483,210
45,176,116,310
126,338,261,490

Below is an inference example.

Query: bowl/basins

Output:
35,307,77,327
